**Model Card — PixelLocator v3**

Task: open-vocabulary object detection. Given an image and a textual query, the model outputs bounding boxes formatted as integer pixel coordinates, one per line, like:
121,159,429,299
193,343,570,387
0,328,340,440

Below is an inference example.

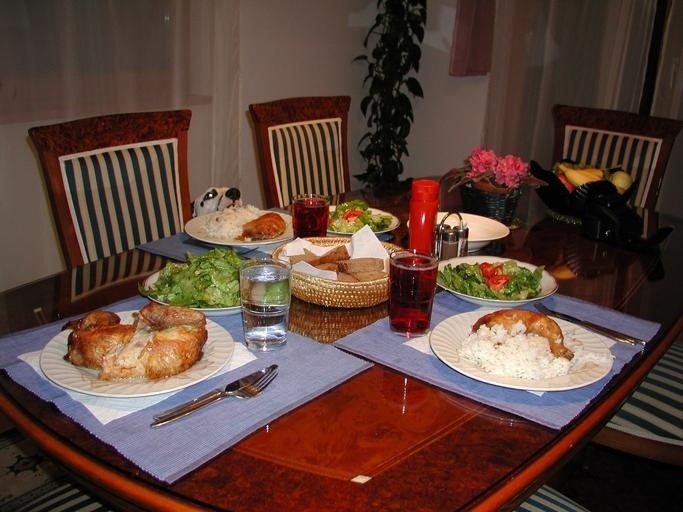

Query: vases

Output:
462,179,518,225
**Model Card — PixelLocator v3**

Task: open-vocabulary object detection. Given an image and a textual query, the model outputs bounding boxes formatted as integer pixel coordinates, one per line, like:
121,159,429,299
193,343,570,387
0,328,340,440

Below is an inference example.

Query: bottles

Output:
435,221,469,259
406,178,441,254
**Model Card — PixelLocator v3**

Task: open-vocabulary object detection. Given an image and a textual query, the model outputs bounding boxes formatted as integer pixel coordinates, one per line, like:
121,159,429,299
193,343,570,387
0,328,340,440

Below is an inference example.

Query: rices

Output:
461,320,600,380
199,204,260,241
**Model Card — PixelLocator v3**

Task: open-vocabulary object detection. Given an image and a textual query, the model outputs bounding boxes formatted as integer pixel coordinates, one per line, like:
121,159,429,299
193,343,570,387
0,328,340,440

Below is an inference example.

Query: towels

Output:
136,225,393,264
334,288,660,430
0,296,373,486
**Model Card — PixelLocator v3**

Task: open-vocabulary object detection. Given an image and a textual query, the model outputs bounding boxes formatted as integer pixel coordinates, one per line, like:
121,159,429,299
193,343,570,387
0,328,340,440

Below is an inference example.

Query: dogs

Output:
191,186,241,217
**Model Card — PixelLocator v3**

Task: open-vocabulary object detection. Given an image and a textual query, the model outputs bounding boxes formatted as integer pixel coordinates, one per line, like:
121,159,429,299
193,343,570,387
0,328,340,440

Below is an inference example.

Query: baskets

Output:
271,235,403,309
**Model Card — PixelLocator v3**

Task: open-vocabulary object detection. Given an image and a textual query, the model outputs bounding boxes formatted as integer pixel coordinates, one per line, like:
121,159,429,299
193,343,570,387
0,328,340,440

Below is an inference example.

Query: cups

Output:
386,249,439,338
236,257,293,355
291,191,330,238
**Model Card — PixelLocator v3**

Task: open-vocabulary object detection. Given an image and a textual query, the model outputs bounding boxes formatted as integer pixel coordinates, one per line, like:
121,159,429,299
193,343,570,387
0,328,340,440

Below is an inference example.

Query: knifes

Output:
152,363,278,422
531,300,646,345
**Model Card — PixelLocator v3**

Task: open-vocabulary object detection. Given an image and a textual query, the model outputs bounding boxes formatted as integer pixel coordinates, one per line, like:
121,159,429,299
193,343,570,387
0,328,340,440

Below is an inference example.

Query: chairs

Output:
592,334,683,466
13,483,122,512
247,94,353,208
552,102,683,212
511,485,592,512
26,108,200,267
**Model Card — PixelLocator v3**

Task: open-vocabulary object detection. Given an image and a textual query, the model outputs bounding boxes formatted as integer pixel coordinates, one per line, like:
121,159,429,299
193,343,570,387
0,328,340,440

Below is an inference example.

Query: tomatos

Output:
486,274,512,293
479,262,497,278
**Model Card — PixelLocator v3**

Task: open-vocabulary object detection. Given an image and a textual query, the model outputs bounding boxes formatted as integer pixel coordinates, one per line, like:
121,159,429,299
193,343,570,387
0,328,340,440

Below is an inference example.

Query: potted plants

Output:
355,0,426,206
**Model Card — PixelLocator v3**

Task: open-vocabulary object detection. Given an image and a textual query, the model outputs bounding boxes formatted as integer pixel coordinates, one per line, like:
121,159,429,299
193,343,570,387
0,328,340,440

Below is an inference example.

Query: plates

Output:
430,309,613,390
323,205,401,236
40,308,233,397
435,254,557,310
143,265,241,318
183,211,294,246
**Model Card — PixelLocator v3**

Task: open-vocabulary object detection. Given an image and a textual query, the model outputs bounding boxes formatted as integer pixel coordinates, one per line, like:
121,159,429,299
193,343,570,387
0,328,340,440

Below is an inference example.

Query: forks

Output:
149,368,277,432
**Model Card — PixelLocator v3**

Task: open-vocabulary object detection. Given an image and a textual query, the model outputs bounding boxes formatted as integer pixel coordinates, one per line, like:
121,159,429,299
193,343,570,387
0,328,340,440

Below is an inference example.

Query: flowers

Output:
440,147,547,204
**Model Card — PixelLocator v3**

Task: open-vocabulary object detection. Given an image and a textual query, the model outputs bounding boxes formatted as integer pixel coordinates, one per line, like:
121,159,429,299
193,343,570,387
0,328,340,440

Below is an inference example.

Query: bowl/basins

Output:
404,213,510,254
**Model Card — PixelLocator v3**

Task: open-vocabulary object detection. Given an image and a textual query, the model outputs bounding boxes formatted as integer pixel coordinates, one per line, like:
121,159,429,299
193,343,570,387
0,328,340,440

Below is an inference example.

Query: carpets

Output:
0,427,71,512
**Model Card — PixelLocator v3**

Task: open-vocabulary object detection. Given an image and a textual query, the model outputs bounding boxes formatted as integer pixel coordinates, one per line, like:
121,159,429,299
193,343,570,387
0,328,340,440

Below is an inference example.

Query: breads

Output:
290,244,388,283
